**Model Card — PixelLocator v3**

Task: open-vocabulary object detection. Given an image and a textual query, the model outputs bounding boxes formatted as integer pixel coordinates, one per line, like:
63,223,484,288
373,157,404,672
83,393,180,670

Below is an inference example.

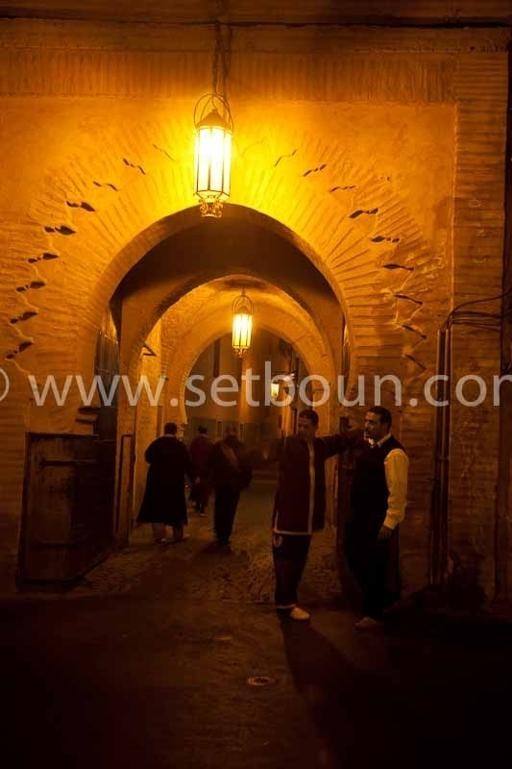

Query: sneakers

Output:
353,618,377,630
277,604,311,620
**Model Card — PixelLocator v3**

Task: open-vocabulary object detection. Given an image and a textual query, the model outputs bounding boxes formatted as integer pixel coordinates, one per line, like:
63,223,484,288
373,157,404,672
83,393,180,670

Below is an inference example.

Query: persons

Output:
137,422,201,544
355,407,410,629
188,425,216,517
272,409,364,621
211,423,251,541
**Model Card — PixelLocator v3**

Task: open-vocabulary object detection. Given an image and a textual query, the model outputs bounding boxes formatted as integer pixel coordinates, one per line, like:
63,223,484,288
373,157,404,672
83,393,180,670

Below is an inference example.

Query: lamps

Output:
190,6,253,360
